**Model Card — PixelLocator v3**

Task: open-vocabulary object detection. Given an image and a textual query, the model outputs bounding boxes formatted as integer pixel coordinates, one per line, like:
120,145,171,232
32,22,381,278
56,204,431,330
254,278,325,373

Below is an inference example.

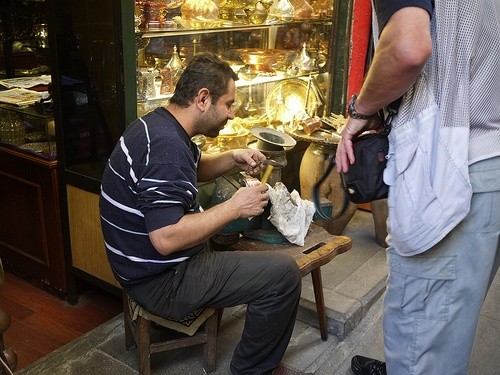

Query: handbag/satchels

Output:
339,128,389,204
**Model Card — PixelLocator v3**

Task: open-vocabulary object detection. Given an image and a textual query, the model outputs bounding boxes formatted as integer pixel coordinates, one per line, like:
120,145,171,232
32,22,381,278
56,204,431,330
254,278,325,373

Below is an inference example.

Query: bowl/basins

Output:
265,78,317,123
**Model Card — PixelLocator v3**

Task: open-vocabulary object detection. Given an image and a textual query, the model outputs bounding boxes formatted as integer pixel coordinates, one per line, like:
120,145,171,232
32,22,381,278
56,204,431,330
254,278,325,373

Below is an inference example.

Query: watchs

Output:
347,94,375,120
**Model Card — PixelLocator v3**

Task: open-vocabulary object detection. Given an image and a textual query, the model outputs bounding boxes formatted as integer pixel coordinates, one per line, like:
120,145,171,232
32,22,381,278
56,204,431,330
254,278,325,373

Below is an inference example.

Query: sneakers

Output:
351,355,387,375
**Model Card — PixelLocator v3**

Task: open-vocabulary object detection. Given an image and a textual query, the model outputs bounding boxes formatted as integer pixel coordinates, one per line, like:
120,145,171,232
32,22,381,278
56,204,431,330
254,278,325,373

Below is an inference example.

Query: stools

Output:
122,284,224,375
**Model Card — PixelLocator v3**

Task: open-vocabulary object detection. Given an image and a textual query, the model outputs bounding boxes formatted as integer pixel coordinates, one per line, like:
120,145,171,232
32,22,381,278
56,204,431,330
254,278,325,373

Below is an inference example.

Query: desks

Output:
284,120,388,249
217,224,352,342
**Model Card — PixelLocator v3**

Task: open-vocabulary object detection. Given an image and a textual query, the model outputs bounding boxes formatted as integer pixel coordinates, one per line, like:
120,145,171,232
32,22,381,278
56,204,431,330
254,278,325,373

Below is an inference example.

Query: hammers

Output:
260,160,284,187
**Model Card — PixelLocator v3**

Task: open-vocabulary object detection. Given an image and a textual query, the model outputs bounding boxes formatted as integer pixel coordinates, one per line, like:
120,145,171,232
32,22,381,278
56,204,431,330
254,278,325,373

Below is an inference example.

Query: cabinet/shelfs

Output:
43,0,353,306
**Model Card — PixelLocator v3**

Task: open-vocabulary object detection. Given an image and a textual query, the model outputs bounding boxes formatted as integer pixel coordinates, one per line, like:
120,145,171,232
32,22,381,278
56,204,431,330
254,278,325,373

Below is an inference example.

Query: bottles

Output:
298,142,358,235
0,110,25,146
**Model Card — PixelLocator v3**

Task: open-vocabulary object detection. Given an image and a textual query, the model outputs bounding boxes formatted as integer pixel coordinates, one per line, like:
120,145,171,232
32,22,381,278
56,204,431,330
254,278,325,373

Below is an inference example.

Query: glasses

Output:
212,92,242,115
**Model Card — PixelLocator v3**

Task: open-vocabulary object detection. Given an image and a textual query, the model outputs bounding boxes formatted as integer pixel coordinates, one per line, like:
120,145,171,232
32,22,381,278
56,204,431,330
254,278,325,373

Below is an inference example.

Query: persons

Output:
336,0,500,375
99,51,302,375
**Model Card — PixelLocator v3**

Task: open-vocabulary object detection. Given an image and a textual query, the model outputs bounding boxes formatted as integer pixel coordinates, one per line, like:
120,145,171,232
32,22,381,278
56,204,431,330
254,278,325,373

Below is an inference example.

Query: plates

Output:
24,131,47,141
17,142,45,152
